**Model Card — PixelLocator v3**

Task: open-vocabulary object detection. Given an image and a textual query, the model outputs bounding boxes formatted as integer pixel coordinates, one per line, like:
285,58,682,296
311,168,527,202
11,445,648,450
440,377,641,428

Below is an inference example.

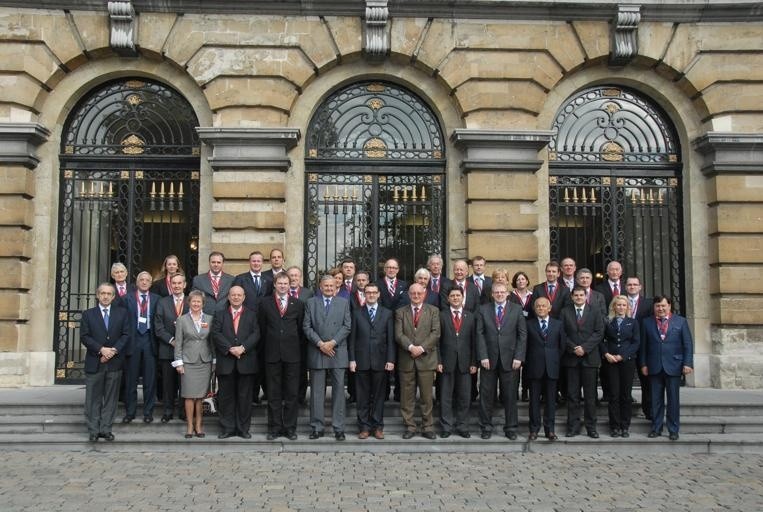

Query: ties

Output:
212,276,220,294
103,308,109,332
412,308,420,327
291,291,297,300
388,279,395,296
456,283,463,288
548,284,556,298
541,319,548,341
630,299,636,320
233,311,239,336
453,310,461,325
612,282,619,296
497,305,503,321
657,318,667,340
369,307,376,325
576,307,582,325
585,291,589,304
476,277,483,297
432,278,438,290
253,274,260,292
176,298,183,317
346,280,351,293
138,294,149,335
277,297,284,312
324,297,331,317
118,286,125,297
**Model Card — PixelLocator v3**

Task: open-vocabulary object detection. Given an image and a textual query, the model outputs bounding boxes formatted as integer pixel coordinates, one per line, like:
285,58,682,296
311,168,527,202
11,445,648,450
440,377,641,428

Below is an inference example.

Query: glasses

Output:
384,266,398,272
626,283,640,288
364,291,380,296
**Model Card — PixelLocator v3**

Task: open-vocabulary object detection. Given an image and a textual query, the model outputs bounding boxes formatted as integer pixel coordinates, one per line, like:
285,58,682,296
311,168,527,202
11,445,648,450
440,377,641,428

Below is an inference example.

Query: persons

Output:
287,266,311,302
210,285,260,438
530,262,571,319
340,260,357,291
192,251,235,317
396,267,439,309
481,267,519,404
230,250,274,404
153,273,189,422
375,258,409,401
347,270,382,403
121,271,162,424
558,257,578,295
525,296,565,440
596,261,626,312
348,283,394,440
622,275,652,419
257,271,304,440
111,262,133,301
312,269,350,306
639,296,694,440
440,260,479,314
511,271,532,402
559,286,604,439
475,283,528,440
599,295,639,438
394,283,441,440
469,255,492,298
80,283,130,442
151,255,189,297
171,290,213,438
436,286,479,438
265,248,286,277
566,268,607,322
427,254,449,295
302,276,351,440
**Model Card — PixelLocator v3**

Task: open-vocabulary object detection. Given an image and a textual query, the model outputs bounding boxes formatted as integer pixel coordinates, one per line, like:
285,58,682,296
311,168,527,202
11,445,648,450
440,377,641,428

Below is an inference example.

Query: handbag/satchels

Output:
208,369,219,397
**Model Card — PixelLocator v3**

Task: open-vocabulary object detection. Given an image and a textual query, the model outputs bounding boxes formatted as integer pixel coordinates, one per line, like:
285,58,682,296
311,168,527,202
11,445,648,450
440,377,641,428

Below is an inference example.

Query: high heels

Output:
185,424,194,439
194,425,205,437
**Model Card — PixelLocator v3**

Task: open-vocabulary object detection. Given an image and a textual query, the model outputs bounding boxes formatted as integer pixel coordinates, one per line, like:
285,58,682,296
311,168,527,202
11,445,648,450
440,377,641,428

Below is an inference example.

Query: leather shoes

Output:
565,431,580,438
218,433,237,439
372,430,384,440
309,430,323,439
179,415,188,420
283,431,298,441
345,396,356,404
506,432,516,441
334,432,345,441
439,431,451,438
588,429,600,441
622,430,630,438
122,414,136,423
358,430,371,439
668,432,680,440
459,431,471,438
479,431,493,439
89,433,100,441
528,432,539,441
143,415,154,422
266,432,283,440
610,429,620,438
238,432,251,440
424,432,436,440
161,414,174,423
100,433,115,440
545,432,559,441
647,431,663,439
401,431,418,439
